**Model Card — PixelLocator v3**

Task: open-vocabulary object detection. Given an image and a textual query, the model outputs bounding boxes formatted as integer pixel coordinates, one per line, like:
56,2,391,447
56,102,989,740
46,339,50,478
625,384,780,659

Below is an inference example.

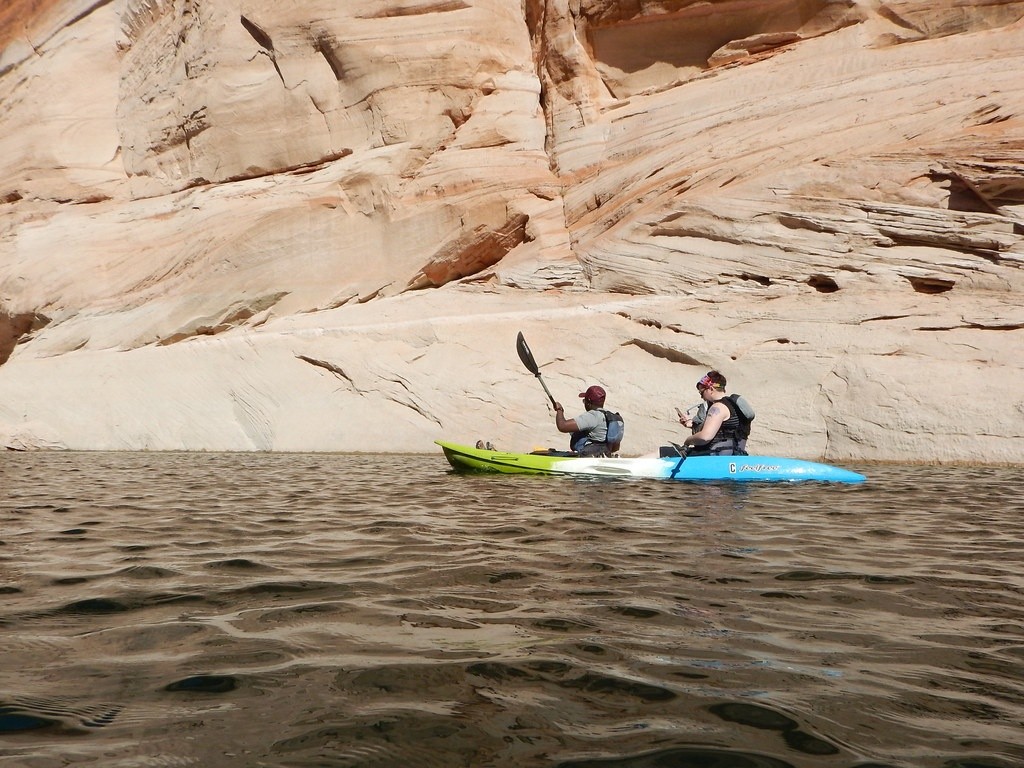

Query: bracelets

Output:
557,407,564,412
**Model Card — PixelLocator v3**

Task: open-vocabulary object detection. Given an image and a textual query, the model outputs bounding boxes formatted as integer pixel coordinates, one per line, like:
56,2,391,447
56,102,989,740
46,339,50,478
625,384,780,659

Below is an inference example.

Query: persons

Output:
640,371,749,459
476,385,612,458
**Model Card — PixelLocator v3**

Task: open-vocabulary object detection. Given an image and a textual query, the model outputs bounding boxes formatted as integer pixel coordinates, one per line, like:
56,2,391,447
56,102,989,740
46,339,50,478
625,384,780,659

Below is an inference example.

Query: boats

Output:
432,438,869,487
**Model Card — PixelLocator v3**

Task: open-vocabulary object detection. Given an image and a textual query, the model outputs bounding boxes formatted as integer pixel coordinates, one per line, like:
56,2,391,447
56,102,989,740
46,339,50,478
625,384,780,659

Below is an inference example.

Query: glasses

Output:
583,399,586,403
699,388,707,396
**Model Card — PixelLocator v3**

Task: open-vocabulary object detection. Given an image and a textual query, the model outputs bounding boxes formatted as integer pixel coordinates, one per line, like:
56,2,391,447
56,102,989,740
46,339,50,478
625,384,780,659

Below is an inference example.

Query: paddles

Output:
667,440,689,460
515,331,575,436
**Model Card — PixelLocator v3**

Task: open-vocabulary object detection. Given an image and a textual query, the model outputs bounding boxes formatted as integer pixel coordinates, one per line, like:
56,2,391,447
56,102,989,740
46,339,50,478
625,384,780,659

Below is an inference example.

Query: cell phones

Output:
675,407,687,423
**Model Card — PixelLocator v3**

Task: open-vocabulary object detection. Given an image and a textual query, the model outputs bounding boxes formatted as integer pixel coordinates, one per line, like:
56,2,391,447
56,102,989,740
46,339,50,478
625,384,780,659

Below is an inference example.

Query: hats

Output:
578,385,606,405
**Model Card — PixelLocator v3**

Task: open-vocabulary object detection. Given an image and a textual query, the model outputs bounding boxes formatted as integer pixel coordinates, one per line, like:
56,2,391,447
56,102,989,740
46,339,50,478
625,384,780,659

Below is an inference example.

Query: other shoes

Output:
615,454,619,457
476,440,486,450
485,441,496,450
602,453,607,457
